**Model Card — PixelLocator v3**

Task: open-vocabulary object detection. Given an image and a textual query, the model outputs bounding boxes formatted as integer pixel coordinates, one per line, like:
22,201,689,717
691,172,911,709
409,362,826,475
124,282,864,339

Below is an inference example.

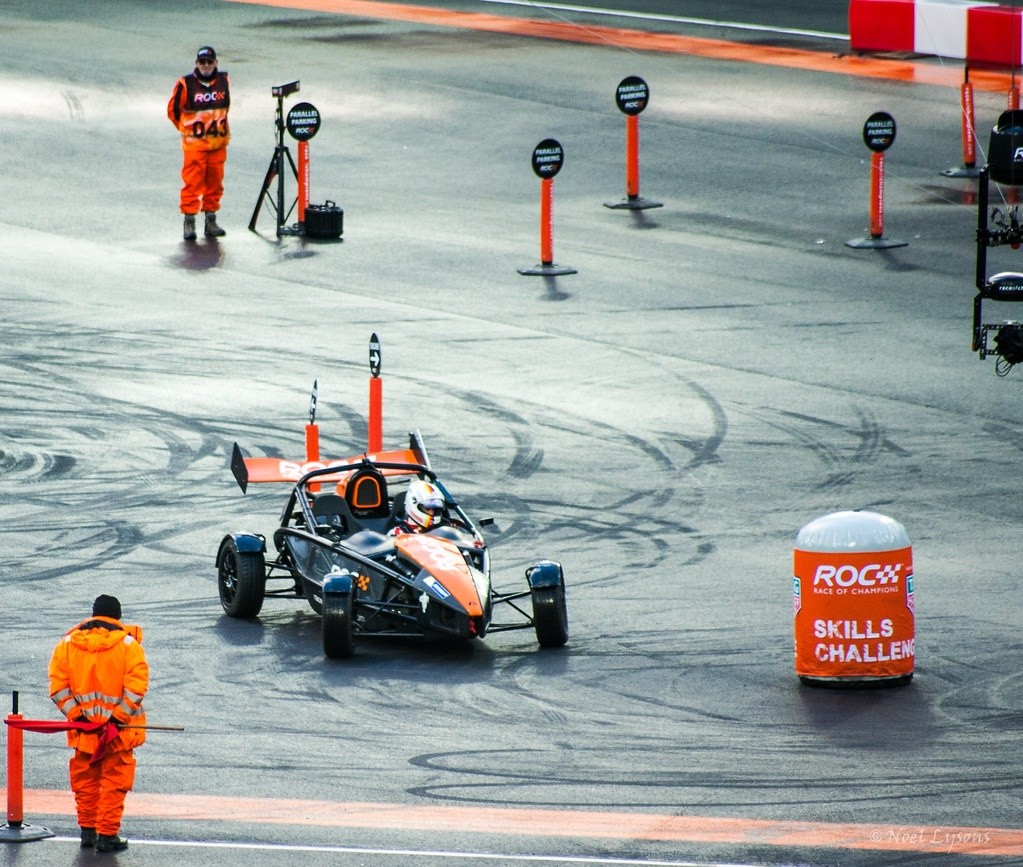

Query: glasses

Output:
198,59,214,65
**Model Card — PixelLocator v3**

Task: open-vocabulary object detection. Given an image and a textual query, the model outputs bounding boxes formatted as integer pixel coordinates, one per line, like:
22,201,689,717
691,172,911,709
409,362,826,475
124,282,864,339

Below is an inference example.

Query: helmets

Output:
405,480,446,528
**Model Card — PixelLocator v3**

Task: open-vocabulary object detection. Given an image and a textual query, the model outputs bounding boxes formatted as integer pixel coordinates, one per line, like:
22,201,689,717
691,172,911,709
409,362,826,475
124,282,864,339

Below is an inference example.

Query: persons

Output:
387,481,482,550
49,594,149,851
167,47,231,240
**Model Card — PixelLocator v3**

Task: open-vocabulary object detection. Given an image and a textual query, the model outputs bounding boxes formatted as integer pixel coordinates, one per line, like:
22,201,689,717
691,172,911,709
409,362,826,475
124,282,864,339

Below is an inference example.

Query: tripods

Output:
247,97,298,240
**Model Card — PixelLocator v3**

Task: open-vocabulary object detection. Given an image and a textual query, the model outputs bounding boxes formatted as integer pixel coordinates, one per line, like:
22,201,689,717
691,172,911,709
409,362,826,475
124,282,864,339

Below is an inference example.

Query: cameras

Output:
272,80,300,97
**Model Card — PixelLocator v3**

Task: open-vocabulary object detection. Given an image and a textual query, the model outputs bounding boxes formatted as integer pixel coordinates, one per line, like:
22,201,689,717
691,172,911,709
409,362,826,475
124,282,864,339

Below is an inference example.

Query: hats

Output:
196,46,216,61
93,595,122,620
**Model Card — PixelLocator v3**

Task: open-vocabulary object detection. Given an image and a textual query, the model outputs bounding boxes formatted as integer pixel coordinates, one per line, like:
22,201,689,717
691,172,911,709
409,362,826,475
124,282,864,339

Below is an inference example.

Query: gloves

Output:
75,714,91,732
99,716,123,736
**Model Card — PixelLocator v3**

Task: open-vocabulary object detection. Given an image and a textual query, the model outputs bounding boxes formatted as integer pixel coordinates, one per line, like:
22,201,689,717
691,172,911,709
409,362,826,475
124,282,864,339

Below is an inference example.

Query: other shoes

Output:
183,216,197,239
97,833,127,852
81,828,97,848
204,214,225,236
